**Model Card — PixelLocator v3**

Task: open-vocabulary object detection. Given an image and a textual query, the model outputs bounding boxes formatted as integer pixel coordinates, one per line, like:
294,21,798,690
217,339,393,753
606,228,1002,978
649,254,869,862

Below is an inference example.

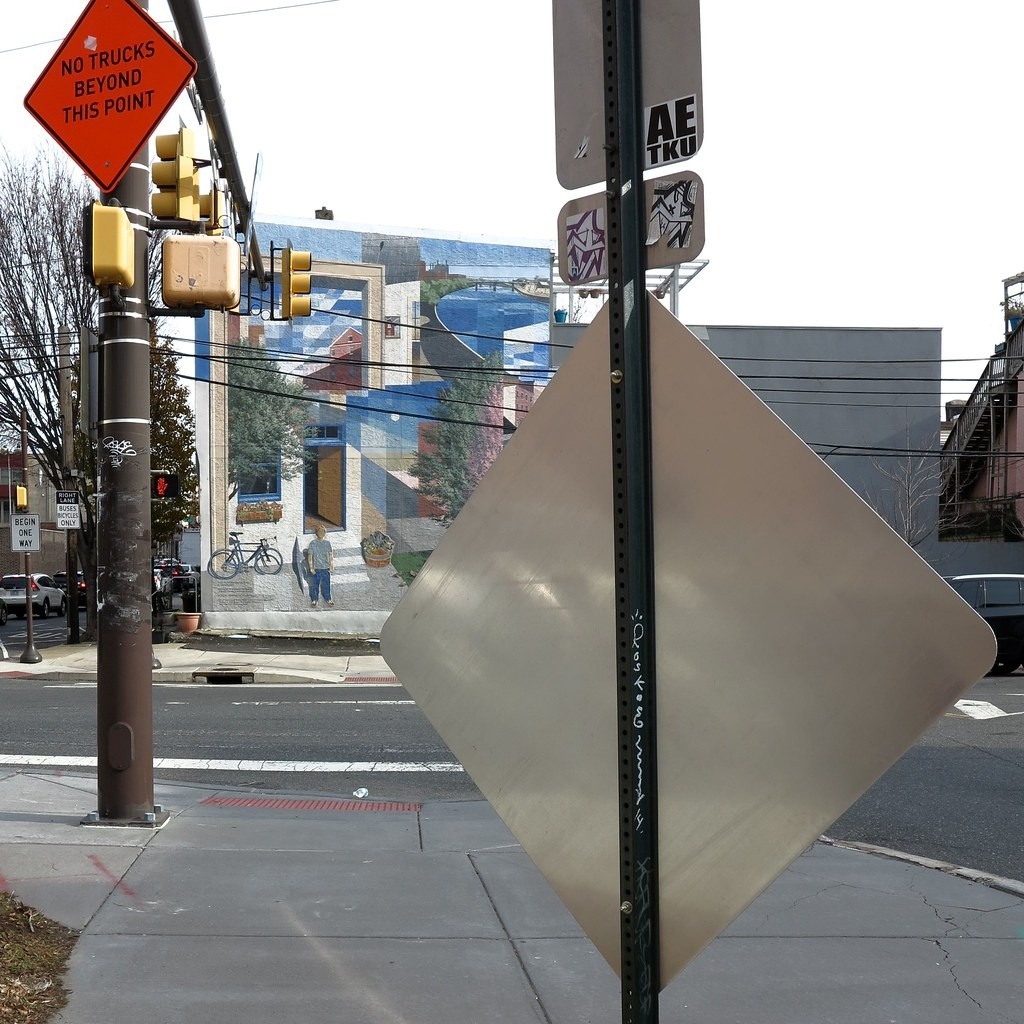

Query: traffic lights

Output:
282,248,313,320
199,187,225,237
151,127,200,222
149,473,181,499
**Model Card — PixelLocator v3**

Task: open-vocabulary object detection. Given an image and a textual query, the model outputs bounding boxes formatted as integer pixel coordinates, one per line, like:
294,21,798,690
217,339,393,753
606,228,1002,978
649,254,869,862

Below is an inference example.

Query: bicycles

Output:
207,531,284,580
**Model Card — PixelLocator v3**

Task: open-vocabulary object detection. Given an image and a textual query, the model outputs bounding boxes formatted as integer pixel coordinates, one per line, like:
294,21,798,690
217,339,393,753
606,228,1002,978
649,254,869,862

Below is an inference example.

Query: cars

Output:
96,558,193,614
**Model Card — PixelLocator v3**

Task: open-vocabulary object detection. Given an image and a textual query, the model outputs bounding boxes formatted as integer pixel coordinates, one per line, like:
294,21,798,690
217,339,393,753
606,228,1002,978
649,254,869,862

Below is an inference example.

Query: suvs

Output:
0,572,68,620
52,570,88,609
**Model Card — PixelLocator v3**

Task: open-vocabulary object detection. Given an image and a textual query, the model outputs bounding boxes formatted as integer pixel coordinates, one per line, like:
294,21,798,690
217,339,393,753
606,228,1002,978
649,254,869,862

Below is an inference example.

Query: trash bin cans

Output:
180,583,201,613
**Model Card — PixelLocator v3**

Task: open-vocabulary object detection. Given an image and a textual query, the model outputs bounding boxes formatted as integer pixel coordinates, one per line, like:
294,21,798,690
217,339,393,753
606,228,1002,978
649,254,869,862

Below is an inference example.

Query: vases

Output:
173,612,201,633
163,612,179,634
